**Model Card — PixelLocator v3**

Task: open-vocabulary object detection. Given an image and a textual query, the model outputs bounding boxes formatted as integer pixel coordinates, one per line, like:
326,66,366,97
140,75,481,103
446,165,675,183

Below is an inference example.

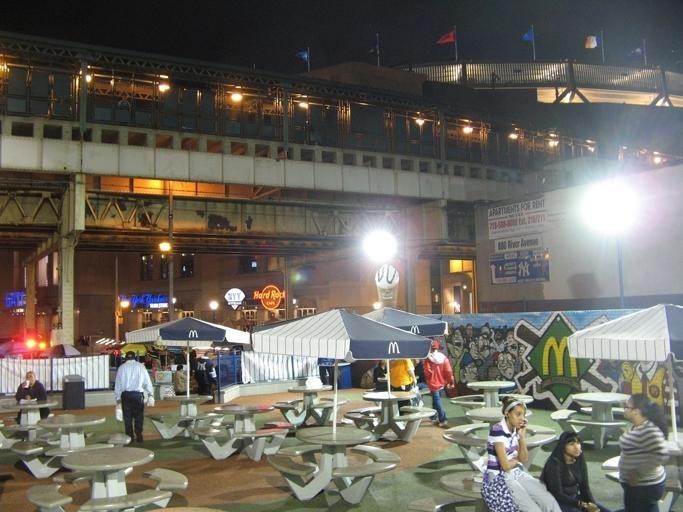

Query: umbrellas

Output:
250,310,435,434
124,316,251,399
566,303,683,440
50,343,81,356
358,307,449,393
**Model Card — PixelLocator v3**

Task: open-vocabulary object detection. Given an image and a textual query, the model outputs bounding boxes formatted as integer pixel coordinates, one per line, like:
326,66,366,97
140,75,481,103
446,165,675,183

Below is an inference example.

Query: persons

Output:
114,349,156,444
193,354,218,395
16,371,51,423
480,397,561,512
116,91,131,112
539,431,612,512
372,339,455,428
172,364,188,396
617,393,670,512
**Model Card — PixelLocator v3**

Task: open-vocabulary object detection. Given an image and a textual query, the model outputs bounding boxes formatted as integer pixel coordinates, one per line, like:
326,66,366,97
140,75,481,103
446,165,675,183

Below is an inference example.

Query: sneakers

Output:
432,418,448,427
137,432,143,442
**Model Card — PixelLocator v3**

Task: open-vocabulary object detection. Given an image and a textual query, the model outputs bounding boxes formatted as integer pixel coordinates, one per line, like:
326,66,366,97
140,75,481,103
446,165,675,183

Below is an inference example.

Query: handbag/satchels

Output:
481,473,520,512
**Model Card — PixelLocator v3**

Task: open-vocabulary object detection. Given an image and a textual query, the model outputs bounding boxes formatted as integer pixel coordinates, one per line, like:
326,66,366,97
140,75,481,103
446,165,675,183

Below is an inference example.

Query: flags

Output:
585,30,604,51
367,35,379,56
625,40,643,58
521,28,534,44
295,50,310,66
436,28,456,43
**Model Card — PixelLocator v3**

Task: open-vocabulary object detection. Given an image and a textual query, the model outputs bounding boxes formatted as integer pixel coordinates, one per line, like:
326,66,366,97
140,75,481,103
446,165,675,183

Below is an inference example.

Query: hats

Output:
432,340,439,349
126,351,135,359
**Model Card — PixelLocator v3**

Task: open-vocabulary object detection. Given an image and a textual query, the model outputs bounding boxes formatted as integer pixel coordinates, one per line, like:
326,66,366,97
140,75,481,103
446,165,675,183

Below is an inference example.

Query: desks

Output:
466,380,516,406
319,363,352,389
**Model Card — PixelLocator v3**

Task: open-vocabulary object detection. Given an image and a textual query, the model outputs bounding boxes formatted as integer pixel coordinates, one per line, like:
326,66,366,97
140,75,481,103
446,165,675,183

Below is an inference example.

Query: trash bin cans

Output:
62,375,86,410
